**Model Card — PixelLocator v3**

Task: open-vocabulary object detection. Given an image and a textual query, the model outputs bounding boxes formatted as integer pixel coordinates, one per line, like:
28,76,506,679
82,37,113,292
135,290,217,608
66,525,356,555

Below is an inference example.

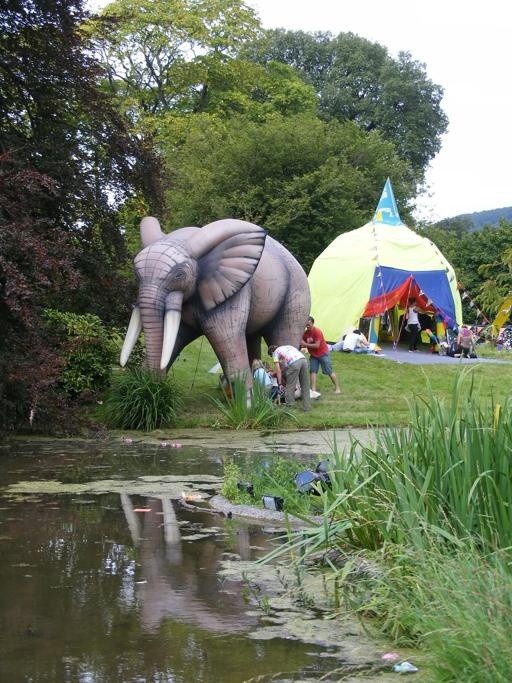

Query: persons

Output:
300,315,340,392
455,324,476,359
252,342,311,408
404,295,427,352
342,329,372,352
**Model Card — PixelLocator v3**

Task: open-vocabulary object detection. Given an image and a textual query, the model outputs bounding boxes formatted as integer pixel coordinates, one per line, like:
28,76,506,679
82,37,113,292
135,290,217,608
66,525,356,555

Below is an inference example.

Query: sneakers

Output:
408,349,421,352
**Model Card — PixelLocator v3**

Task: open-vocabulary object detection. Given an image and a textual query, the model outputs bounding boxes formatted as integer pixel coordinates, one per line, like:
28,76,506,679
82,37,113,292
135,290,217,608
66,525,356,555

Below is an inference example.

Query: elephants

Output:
120,216,311,410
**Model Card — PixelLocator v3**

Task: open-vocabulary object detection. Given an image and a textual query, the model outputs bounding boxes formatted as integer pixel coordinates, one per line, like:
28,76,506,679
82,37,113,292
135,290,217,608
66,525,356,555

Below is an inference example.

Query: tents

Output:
310,174,459,352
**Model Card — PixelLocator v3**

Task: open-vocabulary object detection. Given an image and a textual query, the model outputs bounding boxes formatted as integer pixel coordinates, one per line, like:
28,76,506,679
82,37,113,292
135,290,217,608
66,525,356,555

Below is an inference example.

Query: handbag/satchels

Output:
450,342,461,358
